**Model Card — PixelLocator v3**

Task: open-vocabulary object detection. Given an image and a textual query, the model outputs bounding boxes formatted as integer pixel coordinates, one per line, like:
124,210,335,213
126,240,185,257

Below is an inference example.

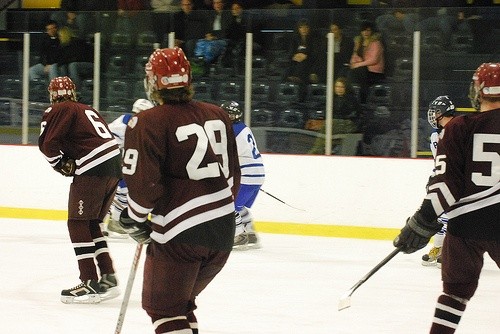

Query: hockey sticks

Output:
113,243,143,334
259,188,307,212
337,245,404,312
60,158,125,210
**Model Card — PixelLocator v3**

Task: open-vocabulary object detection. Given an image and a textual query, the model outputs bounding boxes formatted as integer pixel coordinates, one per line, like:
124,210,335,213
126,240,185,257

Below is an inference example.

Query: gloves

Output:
393,211,444,254
52,155,75,177
119,207,152,244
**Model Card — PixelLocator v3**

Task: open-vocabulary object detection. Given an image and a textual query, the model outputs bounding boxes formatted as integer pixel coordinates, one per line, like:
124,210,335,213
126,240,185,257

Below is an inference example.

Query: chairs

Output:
0,34,443,127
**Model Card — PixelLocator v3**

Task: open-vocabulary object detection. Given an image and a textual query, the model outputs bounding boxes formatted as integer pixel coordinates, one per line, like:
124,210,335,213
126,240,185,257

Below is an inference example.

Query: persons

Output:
306,76,362,156
106,98,155,235
38,74,125,305
30,0,500,89
219,99,266,250
393,62,500,334
118,45,242,334
420,94,459,271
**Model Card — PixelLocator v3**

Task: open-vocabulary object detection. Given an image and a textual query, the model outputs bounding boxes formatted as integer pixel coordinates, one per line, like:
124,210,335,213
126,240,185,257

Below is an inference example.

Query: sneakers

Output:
97,273,119,301
421,247,443,266
61,279,102,304
246,234,258,247
232,231,250,250
102,230,110,239
107,222,128,238
436,256,442,268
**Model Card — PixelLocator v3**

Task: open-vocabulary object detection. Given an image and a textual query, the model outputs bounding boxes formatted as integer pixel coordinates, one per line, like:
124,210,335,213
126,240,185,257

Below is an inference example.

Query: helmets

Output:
427,96,455,130
220,101,241,124
144,48,192,101
47,76,77,102
132,98,154,114
472,62,500,97
